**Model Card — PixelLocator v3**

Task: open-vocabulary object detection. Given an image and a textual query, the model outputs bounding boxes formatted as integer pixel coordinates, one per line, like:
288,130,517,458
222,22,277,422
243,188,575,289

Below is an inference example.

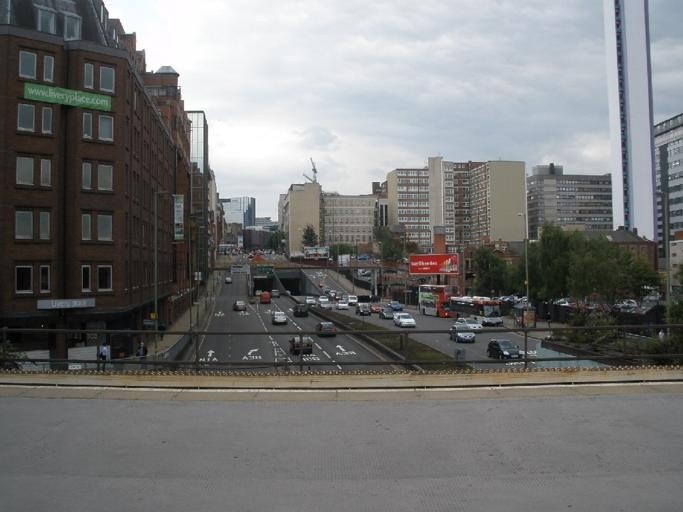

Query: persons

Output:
158,321,166,341
546,312,551,328
100,342,107,372
136,342,147,369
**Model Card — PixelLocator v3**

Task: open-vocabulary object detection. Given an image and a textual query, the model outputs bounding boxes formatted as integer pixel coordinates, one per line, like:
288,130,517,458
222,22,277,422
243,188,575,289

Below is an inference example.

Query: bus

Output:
419,284,459,319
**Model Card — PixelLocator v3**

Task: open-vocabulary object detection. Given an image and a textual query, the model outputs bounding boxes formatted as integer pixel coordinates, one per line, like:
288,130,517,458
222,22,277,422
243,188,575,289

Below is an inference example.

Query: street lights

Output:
517,212,529,303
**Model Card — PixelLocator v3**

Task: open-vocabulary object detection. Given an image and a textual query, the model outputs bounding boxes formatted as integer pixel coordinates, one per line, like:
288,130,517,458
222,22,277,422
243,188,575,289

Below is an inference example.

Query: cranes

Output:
309,156,318,182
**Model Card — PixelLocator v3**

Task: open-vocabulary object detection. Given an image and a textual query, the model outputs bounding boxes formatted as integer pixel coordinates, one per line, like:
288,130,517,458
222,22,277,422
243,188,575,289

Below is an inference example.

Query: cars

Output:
273,311,287,325
234,300,246,311
317,322,337,337
288,336,313,355
225,277,233,283
295,304,309,317
305,271,417,328
256,288,280,304
450,290,663,360
